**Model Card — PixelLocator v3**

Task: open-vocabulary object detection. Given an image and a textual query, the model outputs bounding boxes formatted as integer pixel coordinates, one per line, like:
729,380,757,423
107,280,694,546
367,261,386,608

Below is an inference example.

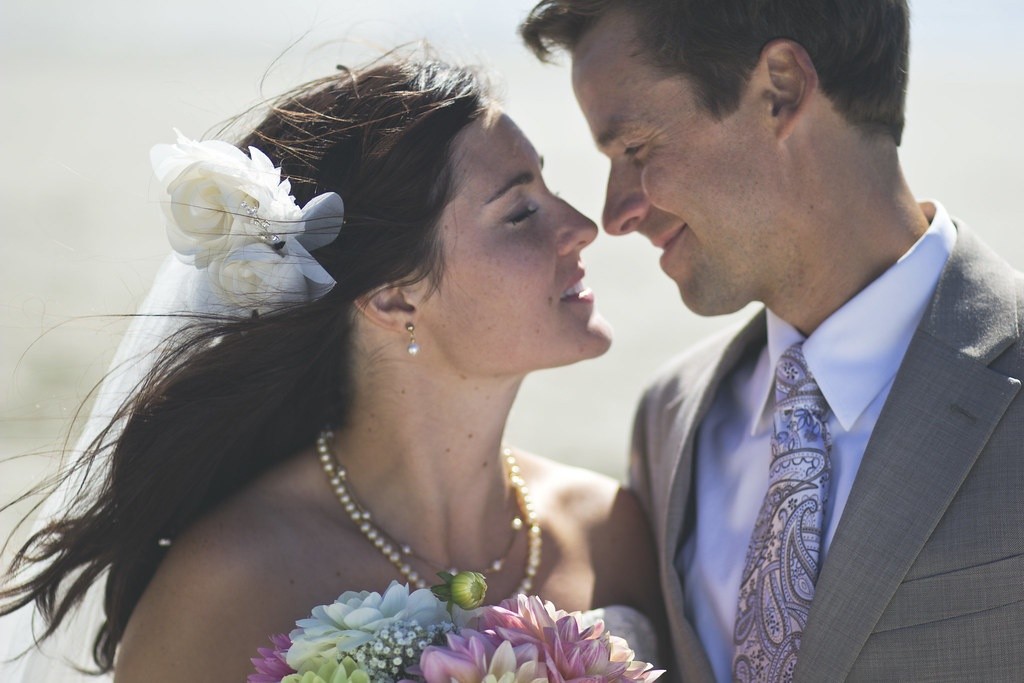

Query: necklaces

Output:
316,425,542,618
327,430,522,575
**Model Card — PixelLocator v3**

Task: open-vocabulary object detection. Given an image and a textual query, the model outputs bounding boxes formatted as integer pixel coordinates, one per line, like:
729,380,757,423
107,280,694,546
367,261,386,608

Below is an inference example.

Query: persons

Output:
0,55,680,683
515,0,1024,682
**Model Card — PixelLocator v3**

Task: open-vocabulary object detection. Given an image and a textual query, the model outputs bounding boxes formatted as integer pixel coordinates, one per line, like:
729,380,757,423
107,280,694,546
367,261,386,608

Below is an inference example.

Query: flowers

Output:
248,583,670,682
152,126,346,316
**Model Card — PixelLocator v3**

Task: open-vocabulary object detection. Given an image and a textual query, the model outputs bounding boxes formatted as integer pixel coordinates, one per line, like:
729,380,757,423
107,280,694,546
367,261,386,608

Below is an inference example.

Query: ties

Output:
730,342,832,683
